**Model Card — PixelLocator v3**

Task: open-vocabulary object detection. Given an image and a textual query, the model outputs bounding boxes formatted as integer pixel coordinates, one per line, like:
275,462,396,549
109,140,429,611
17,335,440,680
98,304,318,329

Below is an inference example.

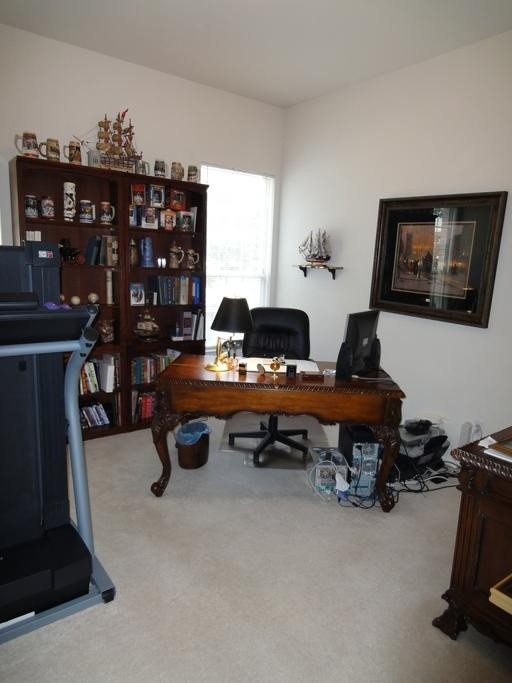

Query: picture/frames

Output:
367,190,509,330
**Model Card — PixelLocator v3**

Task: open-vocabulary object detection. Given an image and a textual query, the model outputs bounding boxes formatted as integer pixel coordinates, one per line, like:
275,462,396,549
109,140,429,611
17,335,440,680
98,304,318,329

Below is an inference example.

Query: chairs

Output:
227,306,310,466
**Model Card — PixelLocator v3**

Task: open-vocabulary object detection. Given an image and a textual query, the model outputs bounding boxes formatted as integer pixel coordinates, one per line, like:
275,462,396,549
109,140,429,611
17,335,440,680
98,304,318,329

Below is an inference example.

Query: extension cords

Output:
386,478,419,486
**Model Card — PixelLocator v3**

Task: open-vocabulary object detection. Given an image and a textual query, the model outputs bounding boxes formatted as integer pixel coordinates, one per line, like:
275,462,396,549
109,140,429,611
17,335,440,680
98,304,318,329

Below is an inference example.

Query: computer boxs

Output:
32,244,60,305
339,423,379,497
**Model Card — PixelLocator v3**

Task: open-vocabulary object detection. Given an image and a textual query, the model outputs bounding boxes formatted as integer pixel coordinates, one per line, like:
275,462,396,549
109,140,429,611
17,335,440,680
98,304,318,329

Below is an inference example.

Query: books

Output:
126,181,197,234
112,234,119,267
84,235,101,265
482,435,512,465
96,235,107,265
78,271,204,432
107,234,112,266
488,574,512,617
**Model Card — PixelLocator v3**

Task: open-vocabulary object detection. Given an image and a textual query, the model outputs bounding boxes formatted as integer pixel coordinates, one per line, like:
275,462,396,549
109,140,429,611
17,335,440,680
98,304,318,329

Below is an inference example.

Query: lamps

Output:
210,295,257,371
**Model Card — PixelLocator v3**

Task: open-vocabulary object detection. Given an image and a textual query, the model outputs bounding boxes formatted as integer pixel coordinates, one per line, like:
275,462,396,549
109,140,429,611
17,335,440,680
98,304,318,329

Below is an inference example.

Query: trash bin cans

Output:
174,421,211,469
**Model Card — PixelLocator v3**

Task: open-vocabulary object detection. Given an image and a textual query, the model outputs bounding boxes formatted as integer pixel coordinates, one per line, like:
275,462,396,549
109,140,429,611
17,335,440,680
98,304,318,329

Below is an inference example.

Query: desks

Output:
150,352,407,513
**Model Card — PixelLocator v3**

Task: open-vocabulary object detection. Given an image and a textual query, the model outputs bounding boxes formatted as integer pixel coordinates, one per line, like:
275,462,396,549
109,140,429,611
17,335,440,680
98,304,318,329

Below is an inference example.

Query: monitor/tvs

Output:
335,309,381,377
0,245,38,311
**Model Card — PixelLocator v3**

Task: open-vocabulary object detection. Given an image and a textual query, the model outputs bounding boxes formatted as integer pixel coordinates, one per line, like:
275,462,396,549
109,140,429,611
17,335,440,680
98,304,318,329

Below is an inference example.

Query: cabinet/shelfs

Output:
8,154,210,442
